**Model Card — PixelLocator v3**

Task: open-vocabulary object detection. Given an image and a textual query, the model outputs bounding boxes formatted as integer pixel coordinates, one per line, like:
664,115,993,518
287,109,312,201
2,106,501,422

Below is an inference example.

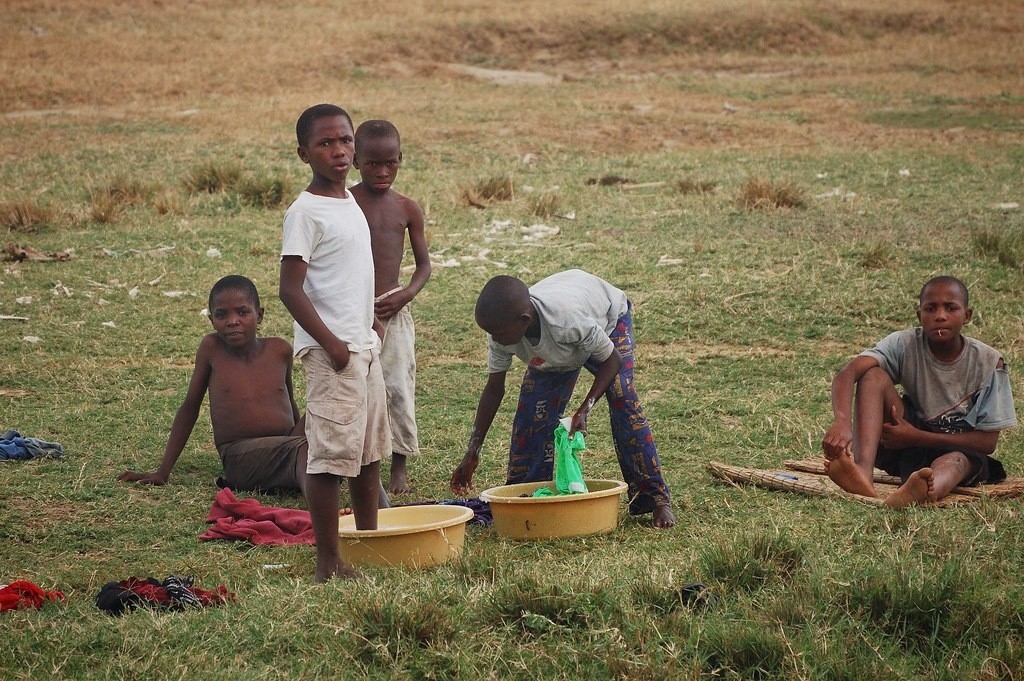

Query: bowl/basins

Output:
482,478,628,541
338,505,475,570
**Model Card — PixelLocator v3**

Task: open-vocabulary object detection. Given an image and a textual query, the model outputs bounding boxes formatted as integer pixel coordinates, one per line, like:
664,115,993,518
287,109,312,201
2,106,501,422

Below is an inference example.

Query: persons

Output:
451,268,676,528
278,103,392,583
117,275,354,519
823,277,1017,512
348,120,432,495
